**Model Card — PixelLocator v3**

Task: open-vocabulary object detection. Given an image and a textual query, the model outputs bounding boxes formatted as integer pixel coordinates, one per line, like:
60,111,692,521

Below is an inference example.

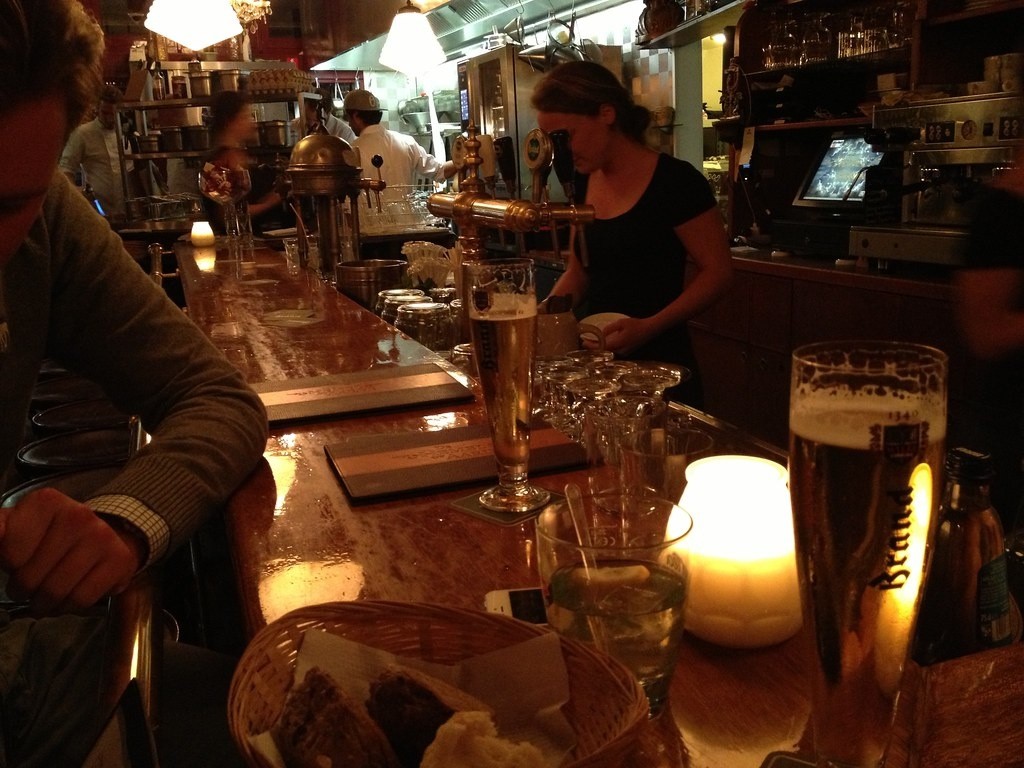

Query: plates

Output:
578,312,632,342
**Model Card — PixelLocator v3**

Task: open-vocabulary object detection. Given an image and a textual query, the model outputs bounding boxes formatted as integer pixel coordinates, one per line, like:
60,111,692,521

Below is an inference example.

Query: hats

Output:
344,88,388,113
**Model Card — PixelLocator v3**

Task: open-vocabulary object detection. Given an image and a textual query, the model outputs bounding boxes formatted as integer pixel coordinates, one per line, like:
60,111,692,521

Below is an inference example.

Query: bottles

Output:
171,76,188,99
686,0,710,21
378,287,478,379
911,444,1013,664
152,67,165,100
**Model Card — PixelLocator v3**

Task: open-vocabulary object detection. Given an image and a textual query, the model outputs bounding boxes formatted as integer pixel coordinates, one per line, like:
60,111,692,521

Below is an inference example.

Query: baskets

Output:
226,596,649,768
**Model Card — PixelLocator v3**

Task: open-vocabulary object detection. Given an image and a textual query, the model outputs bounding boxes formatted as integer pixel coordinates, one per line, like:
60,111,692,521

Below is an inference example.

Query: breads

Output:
269,665,545,768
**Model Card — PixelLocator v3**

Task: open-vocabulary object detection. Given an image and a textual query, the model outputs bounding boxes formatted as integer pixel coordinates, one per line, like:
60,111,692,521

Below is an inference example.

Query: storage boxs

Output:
187,125,211,151
218,68,241,92
157,126,183,152
243,118,286,148
188,71,210,96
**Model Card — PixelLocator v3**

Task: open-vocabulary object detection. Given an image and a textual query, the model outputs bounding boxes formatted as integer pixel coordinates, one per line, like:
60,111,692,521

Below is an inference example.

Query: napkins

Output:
247,631,579,768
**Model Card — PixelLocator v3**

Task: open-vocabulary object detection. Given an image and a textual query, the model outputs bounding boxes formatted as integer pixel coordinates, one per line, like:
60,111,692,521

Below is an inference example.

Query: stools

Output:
0,374,180,661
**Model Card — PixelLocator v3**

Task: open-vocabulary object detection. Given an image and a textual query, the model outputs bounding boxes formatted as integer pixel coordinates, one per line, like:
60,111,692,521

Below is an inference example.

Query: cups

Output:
283,236,317,272
534,495,695,719
223,202,252,246
618,429,712,559
531,350,692,471
533,308,606,354
460,259,548,515
788,345,948,768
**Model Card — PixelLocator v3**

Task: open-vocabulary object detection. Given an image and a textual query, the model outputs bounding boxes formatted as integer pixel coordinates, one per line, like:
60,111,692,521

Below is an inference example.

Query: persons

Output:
344,89,457,202
59,83,170,221
287,88,359,146
0,1,267,607
530,61,733,407
198,90,282,237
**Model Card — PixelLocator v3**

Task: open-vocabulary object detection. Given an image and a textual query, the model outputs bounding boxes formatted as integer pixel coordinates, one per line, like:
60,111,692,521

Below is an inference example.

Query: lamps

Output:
377,0,448,79
144,0,272,58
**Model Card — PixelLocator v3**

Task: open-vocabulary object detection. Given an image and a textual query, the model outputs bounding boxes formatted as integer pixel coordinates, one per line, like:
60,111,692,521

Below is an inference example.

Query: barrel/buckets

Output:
335,259,408,313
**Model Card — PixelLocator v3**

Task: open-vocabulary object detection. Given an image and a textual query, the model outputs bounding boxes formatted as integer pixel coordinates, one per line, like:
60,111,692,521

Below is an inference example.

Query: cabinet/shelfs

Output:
112,92,323,201
727,0,1024,267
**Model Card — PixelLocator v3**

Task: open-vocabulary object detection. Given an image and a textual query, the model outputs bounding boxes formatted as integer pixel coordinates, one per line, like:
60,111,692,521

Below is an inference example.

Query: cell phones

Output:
484,586,549,627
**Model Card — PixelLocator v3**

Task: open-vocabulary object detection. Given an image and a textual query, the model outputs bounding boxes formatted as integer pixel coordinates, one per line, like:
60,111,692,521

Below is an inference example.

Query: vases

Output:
137,135,158,154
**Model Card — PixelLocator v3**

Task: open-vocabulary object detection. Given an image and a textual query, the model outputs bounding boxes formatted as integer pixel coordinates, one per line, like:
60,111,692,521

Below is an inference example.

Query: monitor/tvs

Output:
791,131,891,209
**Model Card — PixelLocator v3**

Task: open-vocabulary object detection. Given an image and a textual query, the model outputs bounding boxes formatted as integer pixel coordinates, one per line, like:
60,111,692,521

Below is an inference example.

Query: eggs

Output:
248,69,314,96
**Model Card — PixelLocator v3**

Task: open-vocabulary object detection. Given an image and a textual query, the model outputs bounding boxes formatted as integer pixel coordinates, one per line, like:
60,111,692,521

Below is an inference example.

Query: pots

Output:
189,71,211,96
210,69,242,95
137,121,287,153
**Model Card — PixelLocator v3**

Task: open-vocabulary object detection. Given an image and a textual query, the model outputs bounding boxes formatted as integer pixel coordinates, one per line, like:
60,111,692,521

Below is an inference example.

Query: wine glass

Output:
761,0,911,71
